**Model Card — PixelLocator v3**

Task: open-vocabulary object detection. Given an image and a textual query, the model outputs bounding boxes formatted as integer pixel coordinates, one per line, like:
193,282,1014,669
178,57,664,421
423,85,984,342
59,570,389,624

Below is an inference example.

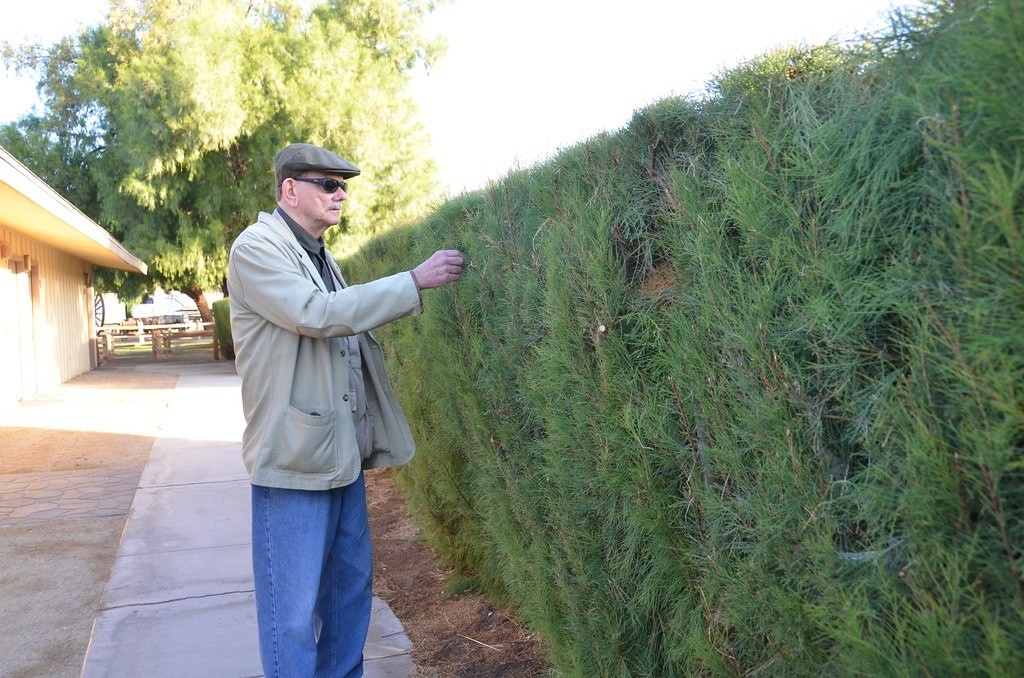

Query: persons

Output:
227,142,463,678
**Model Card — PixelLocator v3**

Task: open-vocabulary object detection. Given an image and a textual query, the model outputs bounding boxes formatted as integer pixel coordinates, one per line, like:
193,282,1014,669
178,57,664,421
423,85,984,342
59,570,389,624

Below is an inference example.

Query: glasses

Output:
282,177,348,193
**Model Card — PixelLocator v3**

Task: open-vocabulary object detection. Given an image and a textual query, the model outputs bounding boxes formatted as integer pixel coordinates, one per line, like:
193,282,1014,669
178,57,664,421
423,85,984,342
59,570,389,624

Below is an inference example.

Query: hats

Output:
274,143,361,188
